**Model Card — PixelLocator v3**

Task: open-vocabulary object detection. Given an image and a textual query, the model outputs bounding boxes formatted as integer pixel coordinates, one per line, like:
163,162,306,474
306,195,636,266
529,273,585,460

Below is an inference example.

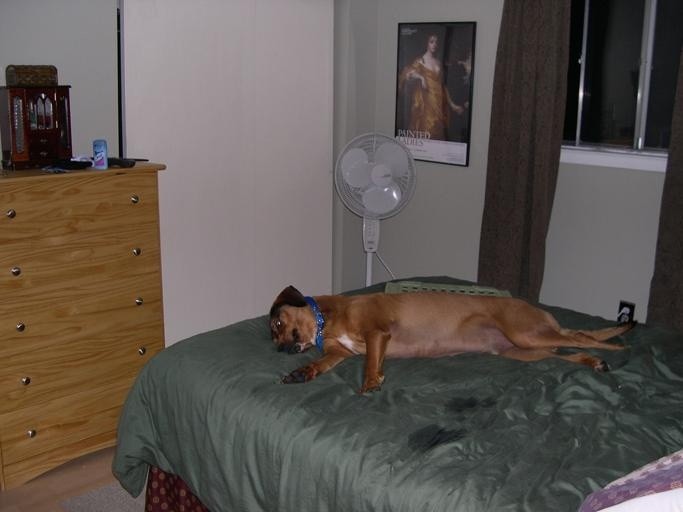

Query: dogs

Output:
268,284,642,397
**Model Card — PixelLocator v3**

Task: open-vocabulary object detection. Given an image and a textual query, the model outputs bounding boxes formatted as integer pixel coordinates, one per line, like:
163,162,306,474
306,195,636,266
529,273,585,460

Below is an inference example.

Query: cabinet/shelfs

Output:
0,159,167,493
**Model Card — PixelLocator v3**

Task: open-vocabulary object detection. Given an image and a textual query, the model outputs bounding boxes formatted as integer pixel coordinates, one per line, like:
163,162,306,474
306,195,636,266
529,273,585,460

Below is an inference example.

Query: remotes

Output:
107,157,134,168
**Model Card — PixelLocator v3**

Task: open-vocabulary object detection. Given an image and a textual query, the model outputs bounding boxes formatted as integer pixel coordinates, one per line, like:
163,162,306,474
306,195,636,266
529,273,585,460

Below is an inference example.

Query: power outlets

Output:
618,299,635,325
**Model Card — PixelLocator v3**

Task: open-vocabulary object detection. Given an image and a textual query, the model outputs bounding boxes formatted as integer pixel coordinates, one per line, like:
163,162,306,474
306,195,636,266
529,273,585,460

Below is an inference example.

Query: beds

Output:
112,275,682,512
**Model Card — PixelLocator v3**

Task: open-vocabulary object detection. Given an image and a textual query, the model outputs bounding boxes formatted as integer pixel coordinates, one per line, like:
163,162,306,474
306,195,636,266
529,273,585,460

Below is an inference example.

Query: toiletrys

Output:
93,140,106,171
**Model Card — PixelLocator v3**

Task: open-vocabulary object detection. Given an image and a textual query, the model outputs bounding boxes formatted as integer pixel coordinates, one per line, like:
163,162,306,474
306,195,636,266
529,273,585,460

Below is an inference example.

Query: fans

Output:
334,133,417,286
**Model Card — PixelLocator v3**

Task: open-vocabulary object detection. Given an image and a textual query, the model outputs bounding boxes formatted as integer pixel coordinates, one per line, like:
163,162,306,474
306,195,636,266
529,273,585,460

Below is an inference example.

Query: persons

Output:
397,32,463,141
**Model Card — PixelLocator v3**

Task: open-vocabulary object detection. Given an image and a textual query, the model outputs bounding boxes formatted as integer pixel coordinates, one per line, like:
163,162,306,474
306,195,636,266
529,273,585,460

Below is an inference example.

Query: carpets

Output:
57,479,146,511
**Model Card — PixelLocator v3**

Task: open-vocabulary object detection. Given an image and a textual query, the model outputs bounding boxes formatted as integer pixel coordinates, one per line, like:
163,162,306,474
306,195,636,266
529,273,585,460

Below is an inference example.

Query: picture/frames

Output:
394,22,476,167
